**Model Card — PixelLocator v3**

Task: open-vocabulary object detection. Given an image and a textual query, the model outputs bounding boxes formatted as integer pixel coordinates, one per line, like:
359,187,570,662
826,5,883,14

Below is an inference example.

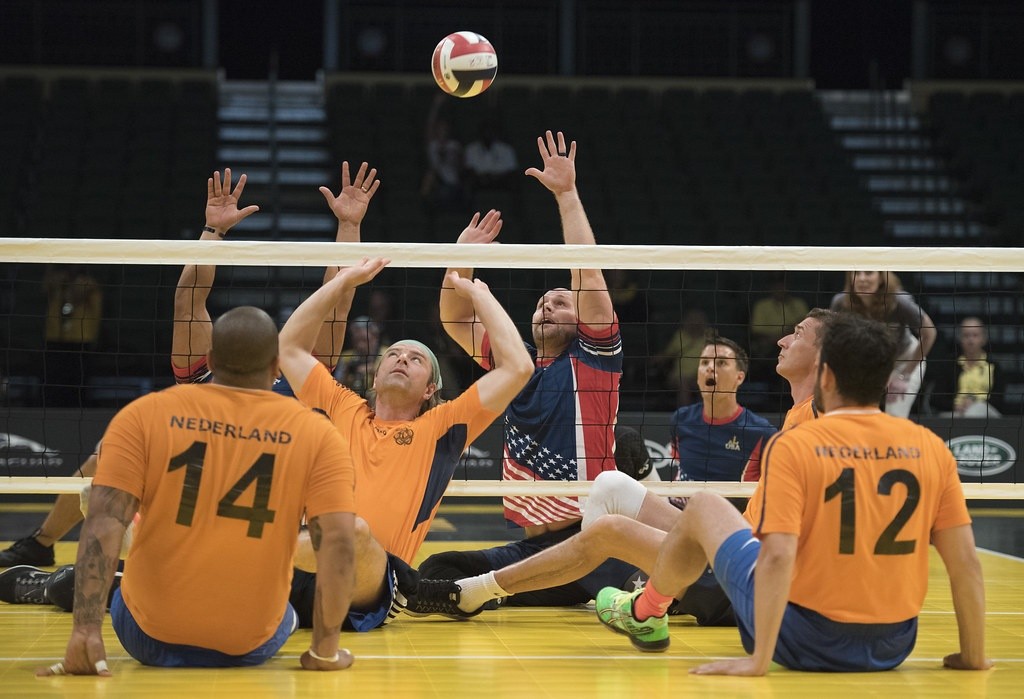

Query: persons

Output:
276,257,536,631
387,268,1006,676
39,258,107,400
387,129,625,604
37,307,355,678
0,160,380,606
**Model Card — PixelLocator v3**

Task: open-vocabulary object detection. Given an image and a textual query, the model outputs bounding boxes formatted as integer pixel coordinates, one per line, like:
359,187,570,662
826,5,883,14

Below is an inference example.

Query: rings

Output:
362,186,367,192
51,663,65,674
559,153,565,156
94,660,109,674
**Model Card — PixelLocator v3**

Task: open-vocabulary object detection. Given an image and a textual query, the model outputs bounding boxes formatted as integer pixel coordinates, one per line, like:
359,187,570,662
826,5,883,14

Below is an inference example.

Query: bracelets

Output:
308,645,339,663
203,226,224,238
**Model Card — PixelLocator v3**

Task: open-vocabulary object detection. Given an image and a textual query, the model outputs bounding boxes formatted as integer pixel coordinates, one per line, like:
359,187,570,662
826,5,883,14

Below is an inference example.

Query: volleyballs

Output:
430,31,499,98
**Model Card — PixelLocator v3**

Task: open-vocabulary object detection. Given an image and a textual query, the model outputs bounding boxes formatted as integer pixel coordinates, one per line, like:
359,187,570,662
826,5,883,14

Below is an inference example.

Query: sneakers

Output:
596,586,665,652
0,538,55,566
403,579,484,619
0,565,51,605
46,565,123,610
